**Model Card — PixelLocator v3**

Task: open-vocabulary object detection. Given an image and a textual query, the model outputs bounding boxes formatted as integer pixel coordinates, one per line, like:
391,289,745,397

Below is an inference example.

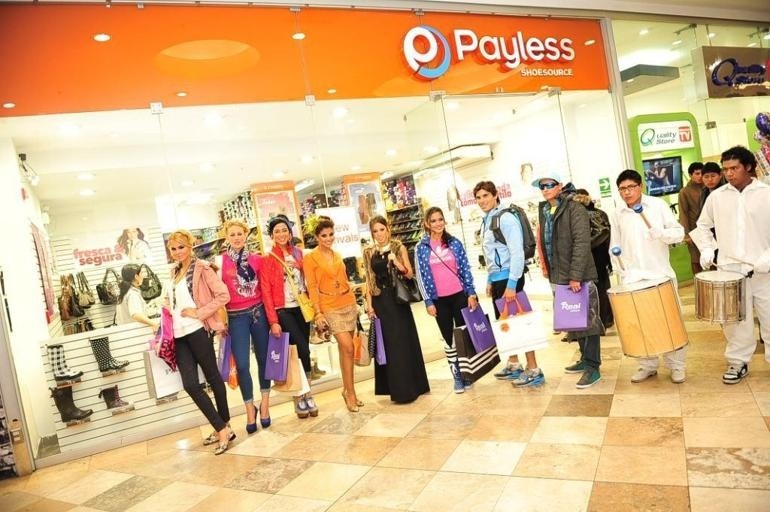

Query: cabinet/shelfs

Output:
191,201,427,319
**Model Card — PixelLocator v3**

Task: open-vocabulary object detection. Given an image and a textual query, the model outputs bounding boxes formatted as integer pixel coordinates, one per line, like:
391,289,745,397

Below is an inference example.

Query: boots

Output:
463,384,472,390
444,346,466,393
89,337,129,372
312,357,326,376
47,345,83,380
49,386,92,422
310,361,321,380
99,385,134,412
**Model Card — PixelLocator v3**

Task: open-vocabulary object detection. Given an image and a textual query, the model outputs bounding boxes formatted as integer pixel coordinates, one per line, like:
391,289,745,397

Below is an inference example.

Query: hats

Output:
531,171,560,187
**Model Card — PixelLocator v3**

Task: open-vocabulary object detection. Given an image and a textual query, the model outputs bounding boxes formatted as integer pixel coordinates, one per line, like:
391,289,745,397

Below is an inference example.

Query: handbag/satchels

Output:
454,314,500,386
296,293,316,322
353,333,370,366
79,291,95,308
567,281,606,343
59,296,73,320
278,359,311,396
271,345,302,391
310,320,332,344
144,349,206,399
104,324,117,328
217,330,232,383
228,354,239,390
215,306,228,329
461,303,496,353
368,316,376,358
156,306,176,373
390,268,423,305
374,315,387,366
491,310,548,360
72,296,84,316
97,279,123,305
554,283,589,331
495,291,532,315
264,325,290,381
139,274,161,300
588,211,610,249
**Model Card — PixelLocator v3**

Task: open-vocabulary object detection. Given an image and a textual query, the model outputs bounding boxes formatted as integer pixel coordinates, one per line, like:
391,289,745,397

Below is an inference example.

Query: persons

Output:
150,229,236,454
439,184,468,225
605,169,689,384
302,216,365,412
115,264,160,335
644,161,670,188
520,162,535,200
532,172,614,388
213,220,273,433
412,207,478,394
260,215,318,418
701,161,732,269
696,146,770,385
363,215,429,403
678,162,717,274
117,228,155,259
473,180,546,388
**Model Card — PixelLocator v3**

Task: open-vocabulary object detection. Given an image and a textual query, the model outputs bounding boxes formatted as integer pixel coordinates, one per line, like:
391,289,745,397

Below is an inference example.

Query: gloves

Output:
699,248,715,271
646,227,663,240
615,264,629,277
753,261,769,274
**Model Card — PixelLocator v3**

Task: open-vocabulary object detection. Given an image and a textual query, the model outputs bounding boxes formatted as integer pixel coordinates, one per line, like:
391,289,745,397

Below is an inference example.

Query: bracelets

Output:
368,311,375,316
313,310,325,317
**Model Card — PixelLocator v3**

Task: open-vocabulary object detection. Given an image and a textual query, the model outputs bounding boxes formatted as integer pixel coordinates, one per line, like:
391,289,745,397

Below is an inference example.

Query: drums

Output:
694,270,747,323
606,276,690,360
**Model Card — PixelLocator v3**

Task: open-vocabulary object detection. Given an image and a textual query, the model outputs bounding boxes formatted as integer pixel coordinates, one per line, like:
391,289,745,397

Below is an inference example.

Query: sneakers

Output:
494,364,524,381
576,367,601,388
722,363,749,384
671,369,686,383
512,367,545,388
631,368,657,382
564,361,584,373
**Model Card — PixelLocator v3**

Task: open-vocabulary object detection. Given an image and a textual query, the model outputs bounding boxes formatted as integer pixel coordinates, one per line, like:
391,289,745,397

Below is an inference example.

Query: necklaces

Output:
174,264,191,286
319,247,331,255
377,242,390,249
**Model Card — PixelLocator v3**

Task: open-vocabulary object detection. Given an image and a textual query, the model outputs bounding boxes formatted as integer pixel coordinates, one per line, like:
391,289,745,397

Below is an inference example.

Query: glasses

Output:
539,182,558,190
618,184,639,193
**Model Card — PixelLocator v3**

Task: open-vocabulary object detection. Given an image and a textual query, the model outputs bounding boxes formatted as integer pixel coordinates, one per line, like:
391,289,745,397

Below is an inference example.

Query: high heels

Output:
247,406,258,434
342,388,364,408
294,396,309,418
304,397,318,417
260,403,270,427
344,389,359,412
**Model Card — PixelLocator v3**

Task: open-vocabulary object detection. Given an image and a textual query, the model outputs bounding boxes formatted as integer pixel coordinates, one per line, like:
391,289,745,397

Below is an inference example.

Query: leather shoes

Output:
214,428,236,455
203,424,231,445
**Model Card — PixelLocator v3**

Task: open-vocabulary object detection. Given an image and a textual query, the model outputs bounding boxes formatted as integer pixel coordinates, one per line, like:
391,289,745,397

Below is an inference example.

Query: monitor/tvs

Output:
642,155,682,197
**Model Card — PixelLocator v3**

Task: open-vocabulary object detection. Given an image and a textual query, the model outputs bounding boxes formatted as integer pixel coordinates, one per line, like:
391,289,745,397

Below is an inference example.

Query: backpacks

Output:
492,204,536,273
